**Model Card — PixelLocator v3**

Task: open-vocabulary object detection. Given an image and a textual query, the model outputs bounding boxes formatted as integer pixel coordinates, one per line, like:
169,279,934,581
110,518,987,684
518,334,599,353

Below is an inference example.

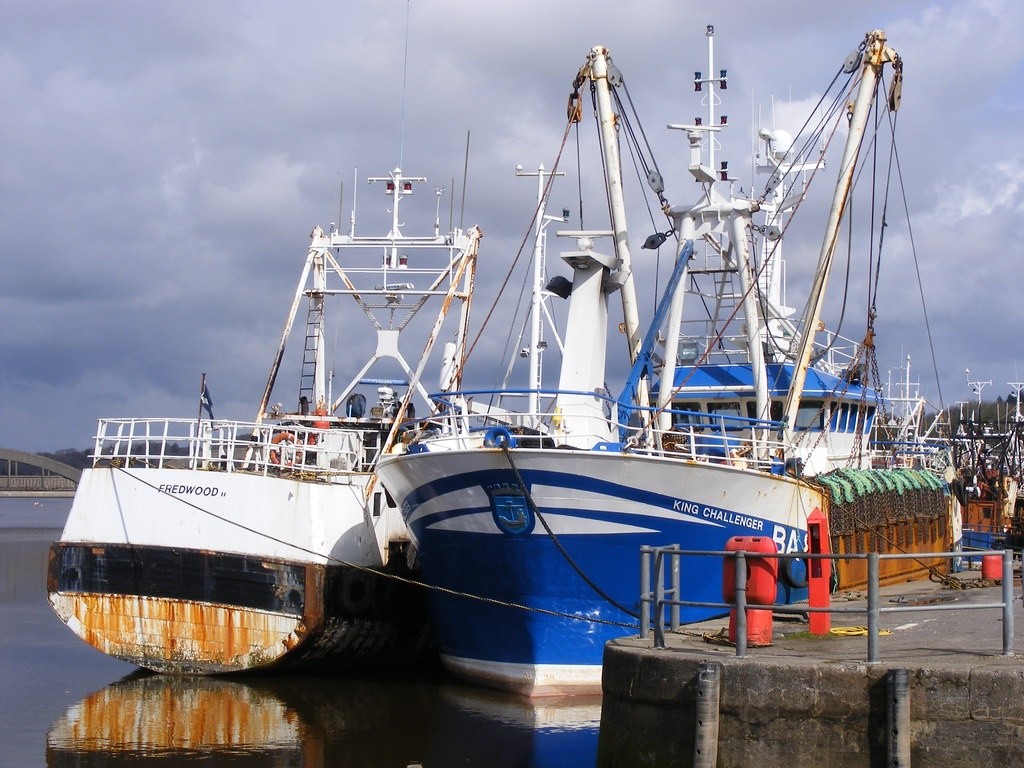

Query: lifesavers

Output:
781,552,809,589
269,432,304,465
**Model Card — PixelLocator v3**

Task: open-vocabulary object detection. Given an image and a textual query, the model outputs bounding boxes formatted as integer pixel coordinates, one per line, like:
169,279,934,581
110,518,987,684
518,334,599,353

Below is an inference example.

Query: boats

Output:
47,0,551,676
373,24,955,695
